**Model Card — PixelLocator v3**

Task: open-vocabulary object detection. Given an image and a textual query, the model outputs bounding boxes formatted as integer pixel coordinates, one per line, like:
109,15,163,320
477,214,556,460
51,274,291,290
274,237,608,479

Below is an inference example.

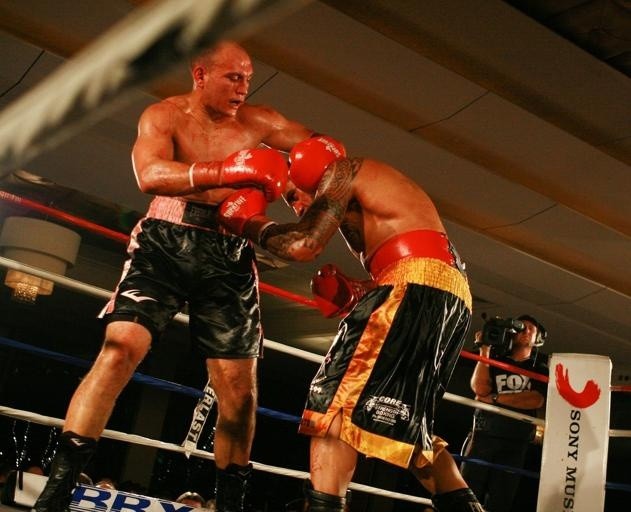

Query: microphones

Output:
527,342,544,347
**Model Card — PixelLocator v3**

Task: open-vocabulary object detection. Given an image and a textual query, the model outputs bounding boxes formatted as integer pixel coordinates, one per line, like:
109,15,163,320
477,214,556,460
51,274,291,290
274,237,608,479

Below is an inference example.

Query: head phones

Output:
535,322,547,348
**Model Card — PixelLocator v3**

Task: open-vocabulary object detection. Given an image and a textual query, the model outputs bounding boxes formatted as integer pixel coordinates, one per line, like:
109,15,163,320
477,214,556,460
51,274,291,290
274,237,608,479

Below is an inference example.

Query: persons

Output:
455,312,548,511
1,456,217,511
30,36,347,511
218,158,485,512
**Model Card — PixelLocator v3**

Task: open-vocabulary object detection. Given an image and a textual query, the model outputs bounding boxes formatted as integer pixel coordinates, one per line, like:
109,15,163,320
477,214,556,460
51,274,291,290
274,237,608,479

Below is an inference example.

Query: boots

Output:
215,460,252,511
32,431,96,512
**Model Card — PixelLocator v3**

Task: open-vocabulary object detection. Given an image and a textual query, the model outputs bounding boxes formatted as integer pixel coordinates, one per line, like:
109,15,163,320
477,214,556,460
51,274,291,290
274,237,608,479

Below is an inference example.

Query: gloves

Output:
189,147,289,200
214,188,275,245
288,131,346,191
311,264,365,320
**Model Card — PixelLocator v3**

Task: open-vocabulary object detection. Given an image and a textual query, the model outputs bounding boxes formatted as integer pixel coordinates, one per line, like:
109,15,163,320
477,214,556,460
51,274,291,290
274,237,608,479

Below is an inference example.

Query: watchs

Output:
492,393,498,405
